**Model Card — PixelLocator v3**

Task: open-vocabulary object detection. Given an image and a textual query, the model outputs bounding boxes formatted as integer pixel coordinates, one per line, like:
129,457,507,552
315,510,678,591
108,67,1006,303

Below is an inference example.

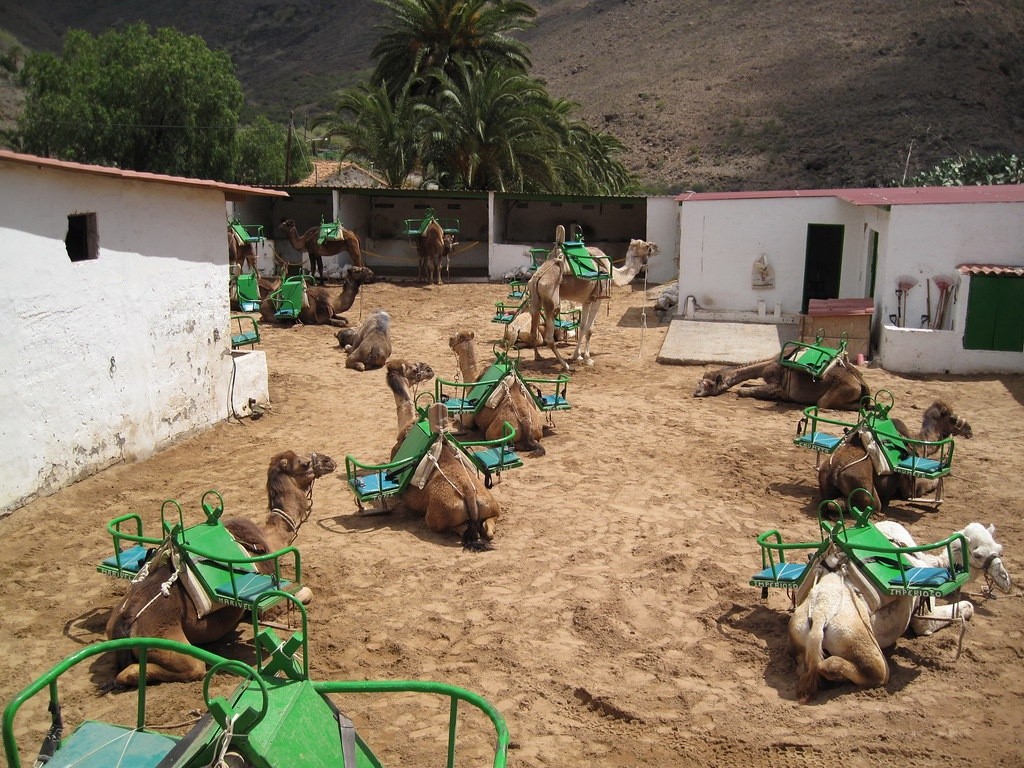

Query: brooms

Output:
930,274,955,330
893,274,920,327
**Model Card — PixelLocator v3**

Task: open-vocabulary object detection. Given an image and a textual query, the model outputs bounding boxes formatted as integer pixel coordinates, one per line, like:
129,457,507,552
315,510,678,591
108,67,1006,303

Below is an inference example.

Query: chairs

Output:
207,626,509,768
508,281,528,299
860,403,955,480
163,503,303,615
230,315,261,350
528,234,582,271
561,234,613,281
0,631,302,768
435,352,519,415
748,505,873,589
417,406,524,489
820,507,970,598
97,504,222,580
490,289,530,324
779,327,849,378
495,351,572,412
344,404,448,503
227,208,460,324
793,404,891,455
525,287,582,331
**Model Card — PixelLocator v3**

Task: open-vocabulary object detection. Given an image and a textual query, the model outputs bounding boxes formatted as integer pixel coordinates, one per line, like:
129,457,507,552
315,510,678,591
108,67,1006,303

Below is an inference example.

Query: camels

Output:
692,343,877,411
333,310,392,372
500,236,662,373
788,518,1011,704
382,358,503,554
447,330,549,459
817,397,973,521
225,220,379,328
417,217,454,287
84,449,338,697
